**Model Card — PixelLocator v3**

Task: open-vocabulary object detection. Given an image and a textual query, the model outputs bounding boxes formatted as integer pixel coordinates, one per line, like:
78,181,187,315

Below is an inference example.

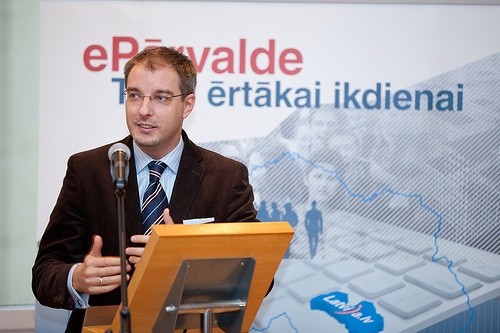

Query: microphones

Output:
108,143,131,189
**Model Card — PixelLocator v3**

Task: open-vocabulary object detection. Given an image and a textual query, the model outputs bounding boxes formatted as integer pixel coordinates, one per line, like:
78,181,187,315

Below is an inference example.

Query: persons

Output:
31,46,275,333
216,105,371,206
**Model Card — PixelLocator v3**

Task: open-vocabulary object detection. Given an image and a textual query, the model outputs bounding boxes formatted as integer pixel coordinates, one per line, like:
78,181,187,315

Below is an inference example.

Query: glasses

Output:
122,88,193,104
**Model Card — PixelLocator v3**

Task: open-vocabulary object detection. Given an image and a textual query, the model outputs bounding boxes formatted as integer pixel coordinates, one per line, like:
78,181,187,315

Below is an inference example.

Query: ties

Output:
141,162,169,235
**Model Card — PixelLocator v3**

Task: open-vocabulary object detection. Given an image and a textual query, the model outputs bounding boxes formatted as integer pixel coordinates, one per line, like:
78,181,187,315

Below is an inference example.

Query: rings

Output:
100,277,103,285
